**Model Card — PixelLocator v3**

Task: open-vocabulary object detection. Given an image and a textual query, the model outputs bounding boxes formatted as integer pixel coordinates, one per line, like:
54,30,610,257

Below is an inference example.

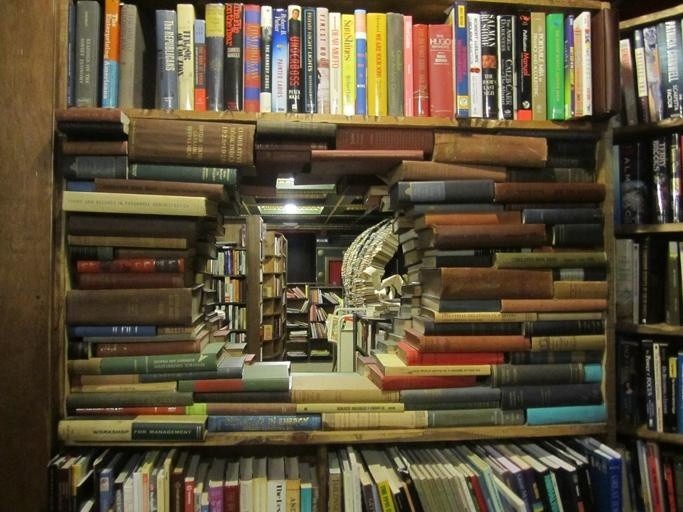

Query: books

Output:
47,436,622,512
68,1,592,120
52,120,609,441
612,19,683,512
